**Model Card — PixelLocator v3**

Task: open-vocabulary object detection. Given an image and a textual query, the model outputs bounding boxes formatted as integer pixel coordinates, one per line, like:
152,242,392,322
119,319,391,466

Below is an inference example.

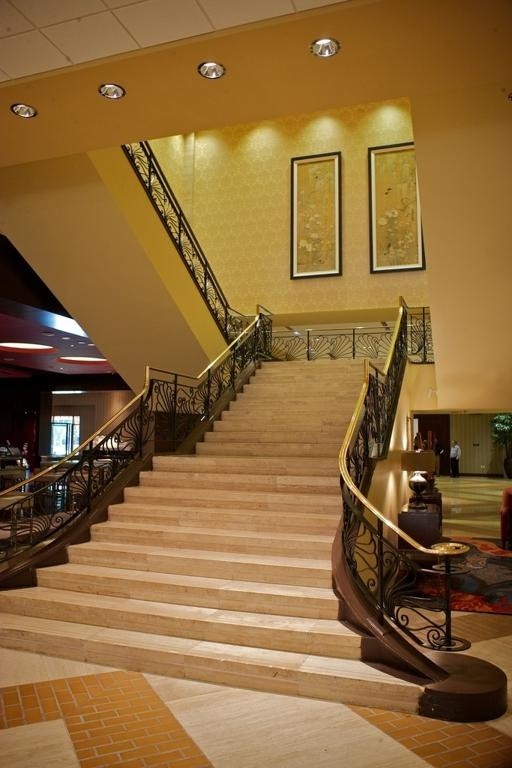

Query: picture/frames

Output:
368,142,425,274
291,152,342,279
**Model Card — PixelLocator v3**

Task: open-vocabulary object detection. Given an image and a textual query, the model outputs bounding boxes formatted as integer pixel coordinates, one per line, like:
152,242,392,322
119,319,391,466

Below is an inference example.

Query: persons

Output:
413,431,444,477
448,440,462,478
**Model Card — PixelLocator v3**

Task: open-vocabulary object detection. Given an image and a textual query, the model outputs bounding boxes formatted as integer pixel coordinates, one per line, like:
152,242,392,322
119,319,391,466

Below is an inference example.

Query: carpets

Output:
383,537,512,615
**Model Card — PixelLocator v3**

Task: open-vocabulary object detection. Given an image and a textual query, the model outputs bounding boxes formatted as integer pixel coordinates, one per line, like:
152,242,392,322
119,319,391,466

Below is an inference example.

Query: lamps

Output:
401,452,435,509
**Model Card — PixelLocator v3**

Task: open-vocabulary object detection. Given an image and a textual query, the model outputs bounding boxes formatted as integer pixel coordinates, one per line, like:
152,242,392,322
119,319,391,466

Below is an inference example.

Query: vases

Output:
504,458,512,479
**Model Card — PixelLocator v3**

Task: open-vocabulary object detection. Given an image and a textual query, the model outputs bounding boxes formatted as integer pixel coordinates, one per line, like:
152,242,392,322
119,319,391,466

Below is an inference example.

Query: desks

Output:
0,491,34,553
398,503,442,549
